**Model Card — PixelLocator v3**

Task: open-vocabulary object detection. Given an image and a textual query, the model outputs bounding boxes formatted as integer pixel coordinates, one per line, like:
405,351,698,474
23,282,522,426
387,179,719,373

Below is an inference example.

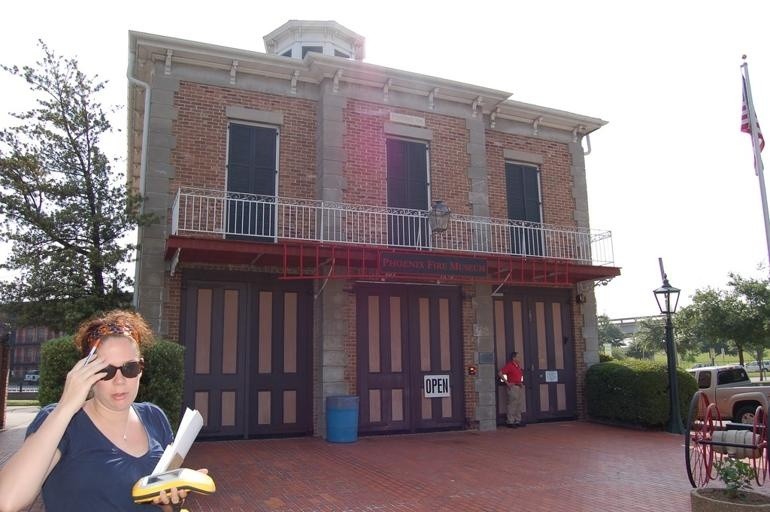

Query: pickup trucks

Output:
683,366,769,432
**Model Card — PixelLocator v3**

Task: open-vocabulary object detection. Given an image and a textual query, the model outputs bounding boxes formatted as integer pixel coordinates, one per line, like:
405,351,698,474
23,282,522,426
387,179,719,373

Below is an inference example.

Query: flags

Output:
738,74,766,179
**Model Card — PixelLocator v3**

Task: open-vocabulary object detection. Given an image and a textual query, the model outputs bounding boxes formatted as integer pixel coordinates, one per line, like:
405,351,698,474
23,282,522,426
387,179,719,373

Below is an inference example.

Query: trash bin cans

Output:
326,395,360,443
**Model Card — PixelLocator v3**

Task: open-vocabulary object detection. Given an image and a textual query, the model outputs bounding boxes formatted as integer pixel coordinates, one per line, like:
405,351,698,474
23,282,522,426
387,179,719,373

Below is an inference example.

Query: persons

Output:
0,305,209,512
496,351,525,428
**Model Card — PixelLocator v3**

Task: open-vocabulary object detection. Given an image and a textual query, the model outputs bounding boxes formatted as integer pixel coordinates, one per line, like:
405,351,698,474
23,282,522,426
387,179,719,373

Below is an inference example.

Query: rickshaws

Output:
683,391,769,492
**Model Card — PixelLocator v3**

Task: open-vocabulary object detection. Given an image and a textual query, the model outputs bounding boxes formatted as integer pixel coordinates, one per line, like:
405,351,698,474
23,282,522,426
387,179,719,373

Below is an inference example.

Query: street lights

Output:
654,272,688,433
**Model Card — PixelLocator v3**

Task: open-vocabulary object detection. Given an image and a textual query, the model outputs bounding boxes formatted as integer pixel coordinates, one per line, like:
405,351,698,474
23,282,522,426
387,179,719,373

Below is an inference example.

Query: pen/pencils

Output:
83,338,100,368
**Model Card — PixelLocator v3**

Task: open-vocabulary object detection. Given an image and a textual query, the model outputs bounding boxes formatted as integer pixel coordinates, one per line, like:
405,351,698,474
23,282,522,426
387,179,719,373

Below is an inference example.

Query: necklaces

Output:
97,403,134,444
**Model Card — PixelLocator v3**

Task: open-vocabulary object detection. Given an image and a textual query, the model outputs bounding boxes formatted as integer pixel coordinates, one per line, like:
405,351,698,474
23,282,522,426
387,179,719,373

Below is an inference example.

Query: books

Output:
149,405,204,476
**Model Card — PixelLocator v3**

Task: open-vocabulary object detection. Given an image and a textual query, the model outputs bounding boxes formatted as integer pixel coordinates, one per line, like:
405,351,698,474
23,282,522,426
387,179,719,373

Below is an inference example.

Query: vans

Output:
745,360,770,372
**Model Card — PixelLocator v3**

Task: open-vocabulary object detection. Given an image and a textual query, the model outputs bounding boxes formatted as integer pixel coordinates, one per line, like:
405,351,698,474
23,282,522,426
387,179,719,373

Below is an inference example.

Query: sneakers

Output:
507,423,524,428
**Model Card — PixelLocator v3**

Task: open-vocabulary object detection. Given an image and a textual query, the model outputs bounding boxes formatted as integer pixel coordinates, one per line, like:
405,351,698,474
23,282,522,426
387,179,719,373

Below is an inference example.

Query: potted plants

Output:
691,453,769,512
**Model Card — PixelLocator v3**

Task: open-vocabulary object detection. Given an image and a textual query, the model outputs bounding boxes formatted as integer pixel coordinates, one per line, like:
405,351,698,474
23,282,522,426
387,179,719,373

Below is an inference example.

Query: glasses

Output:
96,361,140,381
